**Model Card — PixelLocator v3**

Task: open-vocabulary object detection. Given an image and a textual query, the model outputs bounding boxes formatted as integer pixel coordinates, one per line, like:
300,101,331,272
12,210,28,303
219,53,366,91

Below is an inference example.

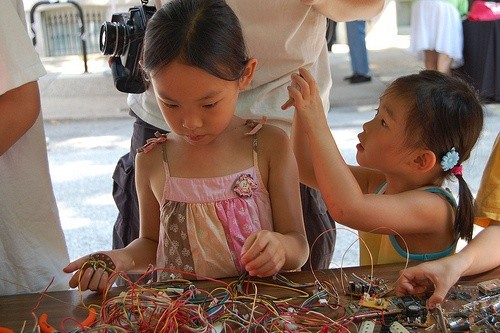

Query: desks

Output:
0,260,499,333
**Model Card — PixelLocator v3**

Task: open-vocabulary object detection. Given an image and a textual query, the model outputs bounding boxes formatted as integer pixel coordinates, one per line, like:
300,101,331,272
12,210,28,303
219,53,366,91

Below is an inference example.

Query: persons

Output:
401,0,474,78
111,0,345,294
336,18,377,85
62,0,388,297
395,128,500,309
0,0,77,297
281,63,487,274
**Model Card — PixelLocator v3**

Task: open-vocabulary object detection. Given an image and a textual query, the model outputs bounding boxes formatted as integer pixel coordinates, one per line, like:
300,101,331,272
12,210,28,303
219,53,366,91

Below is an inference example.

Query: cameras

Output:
99,5,158,94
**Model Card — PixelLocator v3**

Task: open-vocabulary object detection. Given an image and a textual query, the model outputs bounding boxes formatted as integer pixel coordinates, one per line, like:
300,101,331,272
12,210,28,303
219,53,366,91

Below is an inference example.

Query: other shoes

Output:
349,74,372,83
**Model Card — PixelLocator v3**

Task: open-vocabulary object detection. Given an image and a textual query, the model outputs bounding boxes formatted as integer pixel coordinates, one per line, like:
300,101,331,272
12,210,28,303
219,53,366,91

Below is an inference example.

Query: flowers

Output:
441,147,459,171
